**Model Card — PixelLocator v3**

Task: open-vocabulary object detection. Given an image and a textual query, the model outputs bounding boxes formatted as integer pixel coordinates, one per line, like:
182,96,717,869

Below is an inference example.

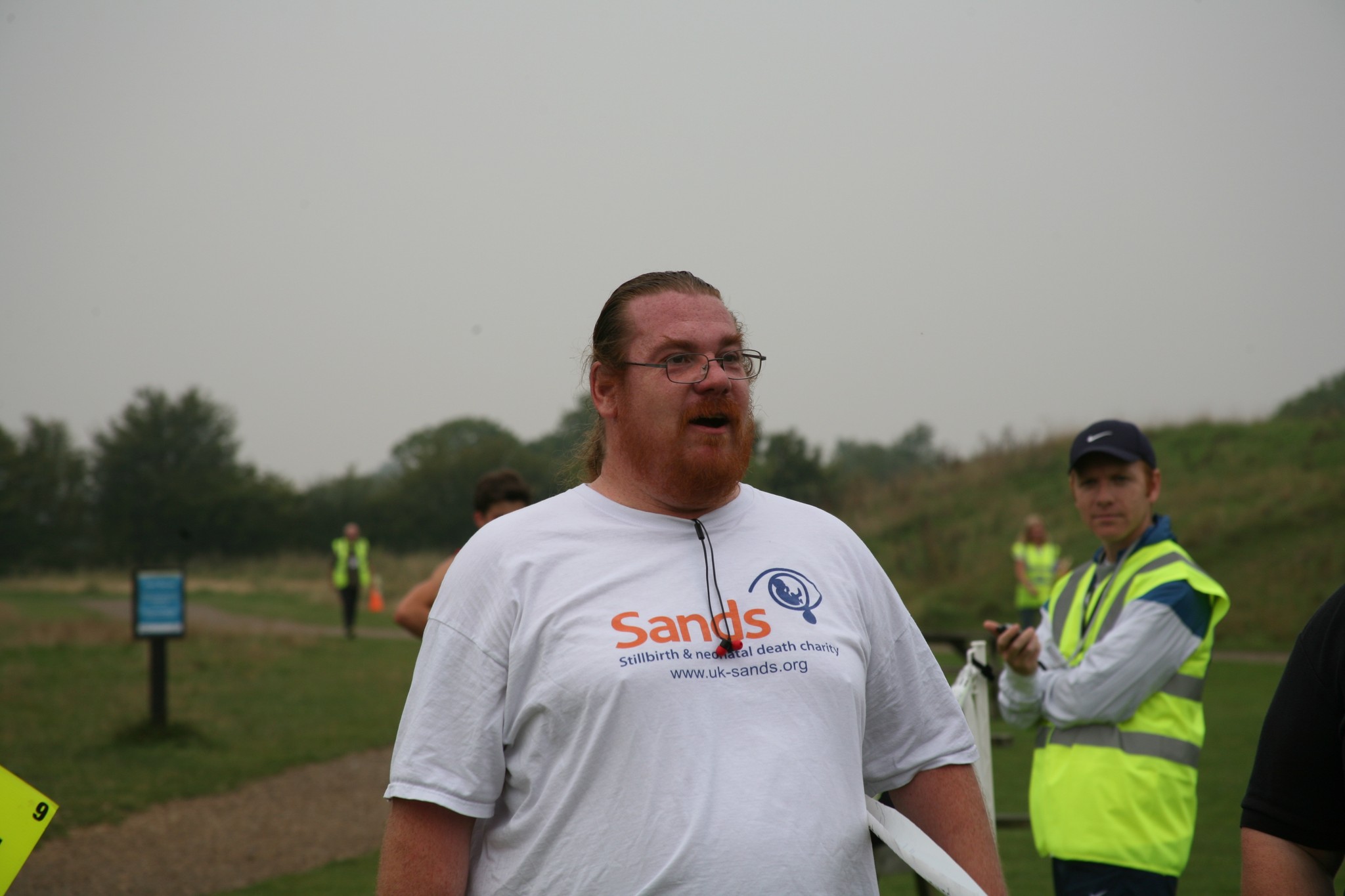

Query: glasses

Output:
615,348,766,383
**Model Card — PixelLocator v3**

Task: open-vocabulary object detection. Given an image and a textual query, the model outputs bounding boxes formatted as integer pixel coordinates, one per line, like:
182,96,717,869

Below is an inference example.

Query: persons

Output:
1240,583,1345,896
377,268,1005,896
395,469,536,636
1010,515,1063,628
331,523,373,626
982,420,1231,896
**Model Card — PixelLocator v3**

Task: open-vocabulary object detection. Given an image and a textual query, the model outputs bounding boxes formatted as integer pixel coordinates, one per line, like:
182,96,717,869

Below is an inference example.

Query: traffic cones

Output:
367,583,385,612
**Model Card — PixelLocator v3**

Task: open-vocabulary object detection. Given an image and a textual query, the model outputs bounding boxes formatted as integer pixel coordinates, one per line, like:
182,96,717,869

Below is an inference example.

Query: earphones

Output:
715,638,743,656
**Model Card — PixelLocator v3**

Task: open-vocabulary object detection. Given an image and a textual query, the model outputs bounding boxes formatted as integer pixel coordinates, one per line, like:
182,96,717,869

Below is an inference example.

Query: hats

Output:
1067,420,1156,468
